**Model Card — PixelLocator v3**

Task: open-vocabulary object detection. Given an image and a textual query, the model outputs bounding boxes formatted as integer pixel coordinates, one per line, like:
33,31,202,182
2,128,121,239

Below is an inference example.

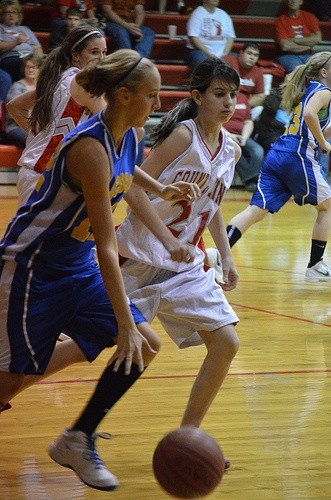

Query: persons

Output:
0,49,202,491
0,58,243,472
0,0,331,500
206,51,331,287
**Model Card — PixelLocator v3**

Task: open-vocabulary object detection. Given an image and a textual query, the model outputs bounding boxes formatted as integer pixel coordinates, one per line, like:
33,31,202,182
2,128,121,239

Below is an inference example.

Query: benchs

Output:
0,0,331,184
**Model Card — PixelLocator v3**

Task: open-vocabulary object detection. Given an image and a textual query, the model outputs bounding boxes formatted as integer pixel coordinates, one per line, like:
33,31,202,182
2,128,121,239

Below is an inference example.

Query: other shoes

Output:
245,182,257,192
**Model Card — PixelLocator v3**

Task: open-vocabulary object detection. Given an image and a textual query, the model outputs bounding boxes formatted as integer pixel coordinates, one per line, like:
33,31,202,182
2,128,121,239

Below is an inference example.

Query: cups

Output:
262,73,273,95
167,25,177,40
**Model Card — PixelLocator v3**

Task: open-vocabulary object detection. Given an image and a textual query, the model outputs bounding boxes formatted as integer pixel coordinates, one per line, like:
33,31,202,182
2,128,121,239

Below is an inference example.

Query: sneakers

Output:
304,258,331,282
206,248,227,286
47,428,120,491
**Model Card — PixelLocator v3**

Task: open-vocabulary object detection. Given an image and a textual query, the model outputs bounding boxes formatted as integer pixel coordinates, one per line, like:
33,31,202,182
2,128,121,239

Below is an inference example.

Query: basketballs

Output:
154,429,225,498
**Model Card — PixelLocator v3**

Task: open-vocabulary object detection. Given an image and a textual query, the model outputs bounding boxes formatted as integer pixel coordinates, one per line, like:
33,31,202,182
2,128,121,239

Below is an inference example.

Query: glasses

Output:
5,11,16,15
